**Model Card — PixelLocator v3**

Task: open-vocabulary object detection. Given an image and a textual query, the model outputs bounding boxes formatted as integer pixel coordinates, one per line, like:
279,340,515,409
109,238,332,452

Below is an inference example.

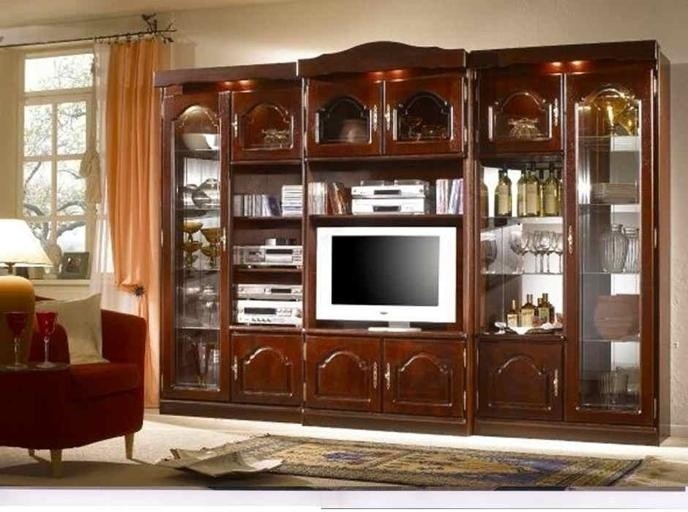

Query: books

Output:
436,177,464,216
233,180,348,220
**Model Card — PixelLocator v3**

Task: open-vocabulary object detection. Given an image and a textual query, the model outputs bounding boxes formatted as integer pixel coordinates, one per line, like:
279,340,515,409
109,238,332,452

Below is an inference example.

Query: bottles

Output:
495,159,563,216
506,292,555,329
595,220,640,272
479,168,489,217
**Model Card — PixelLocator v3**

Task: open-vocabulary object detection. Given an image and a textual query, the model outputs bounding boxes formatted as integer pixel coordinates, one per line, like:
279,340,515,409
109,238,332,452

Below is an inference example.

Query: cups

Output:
585,95,638,135
401,115,447,140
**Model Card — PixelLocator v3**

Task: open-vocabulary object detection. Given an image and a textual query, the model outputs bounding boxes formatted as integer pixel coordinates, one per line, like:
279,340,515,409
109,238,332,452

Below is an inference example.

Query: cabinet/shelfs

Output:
153,41,670,446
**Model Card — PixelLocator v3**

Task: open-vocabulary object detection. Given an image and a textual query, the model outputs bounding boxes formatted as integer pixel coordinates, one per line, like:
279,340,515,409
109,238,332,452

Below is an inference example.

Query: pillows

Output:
35,292,110,365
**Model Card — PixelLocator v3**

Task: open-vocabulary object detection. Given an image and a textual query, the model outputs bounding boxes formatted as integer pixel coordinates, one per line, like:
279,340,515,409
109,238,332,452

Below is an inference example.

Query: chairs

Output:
0,296,146,477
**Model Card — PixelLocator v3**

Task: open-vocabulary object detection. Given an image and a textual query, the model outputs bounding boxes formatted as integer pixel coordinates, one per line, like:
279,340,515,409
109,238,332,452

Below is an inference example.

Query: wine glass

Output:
35,310,58,369
181,222,220,268
480,239,500,274
509,230,564,275
5,311,32,369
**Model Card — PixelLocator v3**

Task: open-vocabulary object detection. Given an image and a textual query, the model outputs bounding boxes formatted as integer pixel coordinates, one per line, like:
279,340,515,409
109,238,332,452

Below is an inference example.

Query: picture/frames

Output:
59,253,93,278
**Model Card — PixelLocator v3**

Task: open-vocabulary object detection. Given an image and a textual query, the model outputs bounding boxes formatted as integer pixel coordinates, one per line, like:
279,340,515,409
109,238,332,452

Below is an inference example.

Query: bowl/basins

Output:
589,182,640,204
180,132,218,150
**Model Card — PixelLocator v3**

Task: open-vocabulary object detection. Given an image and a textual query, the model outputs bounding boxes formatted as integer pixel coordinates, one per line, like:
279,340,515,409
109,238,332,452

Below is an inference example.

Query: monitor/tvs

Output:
316,226,456,332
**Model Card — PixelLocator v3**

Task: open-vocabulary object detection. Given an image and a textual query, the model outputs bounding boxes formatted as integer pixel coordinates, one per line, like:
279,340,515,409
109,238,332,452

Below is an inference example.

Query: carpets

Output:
173,435,640,491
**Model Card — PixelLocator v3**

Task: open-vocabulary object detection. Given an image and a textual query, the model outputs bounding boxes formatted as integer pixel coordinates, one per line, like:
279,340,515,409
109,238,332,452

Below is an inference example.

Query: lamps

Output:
0,219,54,361
